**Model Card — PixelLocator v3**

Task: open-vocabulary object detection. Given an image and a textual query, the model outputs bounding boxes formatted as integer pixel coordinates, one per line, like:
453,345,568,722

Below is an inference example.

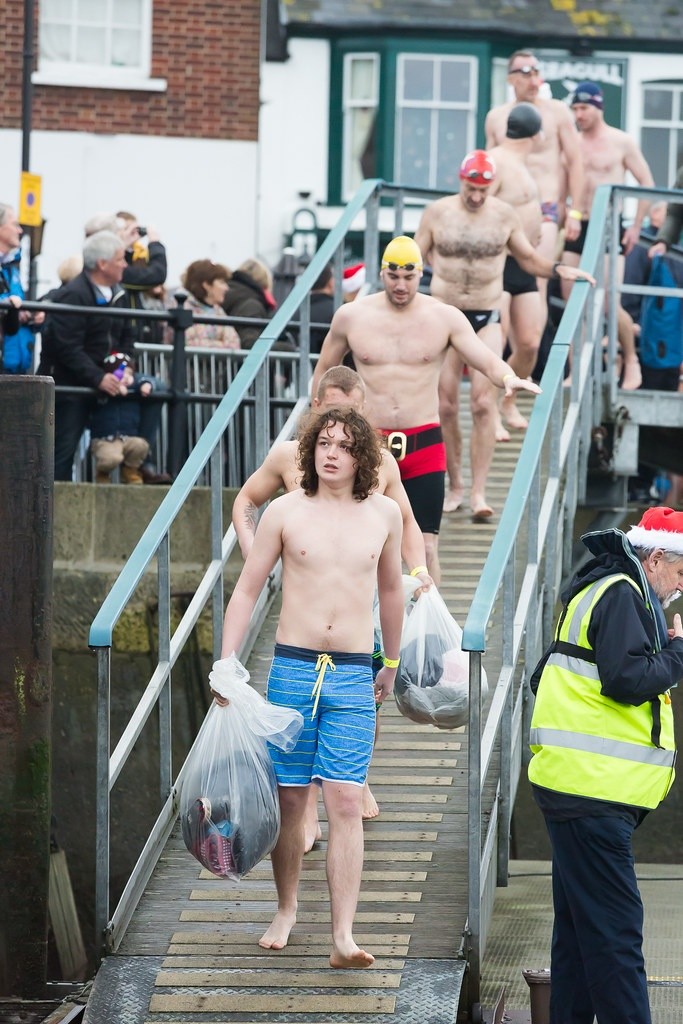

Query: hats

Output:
507,103,542,140
460,150,494,184
572,83,604,111
624,508,683,555
103,352,134,375
381,235,423,272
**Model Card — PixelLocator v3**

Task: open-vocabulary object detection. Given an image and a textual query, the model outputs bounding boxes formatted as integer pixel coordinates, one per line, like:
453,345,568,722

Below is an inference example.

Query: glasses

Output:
509,64,538,74
388,262,416,272
467,171,491,181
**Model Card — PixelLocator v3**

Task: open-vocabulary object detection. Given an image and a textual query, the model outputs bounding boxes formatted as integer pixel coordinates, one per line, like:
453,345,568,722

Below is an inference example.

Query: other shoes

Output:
123,465,143,485
142,463,171,483
95,471,110,484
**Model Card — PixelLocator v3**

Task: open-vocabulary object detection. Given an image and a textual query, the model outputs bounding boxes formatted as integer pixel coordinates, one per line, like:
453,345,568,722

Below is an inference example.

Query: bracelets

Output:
384,658,400,668
553,264,560,273
410,567,427,576
569,210,582,221
503,375,512,382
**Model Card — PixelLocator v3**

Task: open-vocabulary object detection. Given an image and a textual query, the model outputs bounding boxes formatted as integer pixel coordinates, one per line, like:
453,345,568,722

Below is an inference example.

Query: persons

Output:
559,83,656,392
232,365,434,852
414,150,596,518
647,166,683,257
486,102,541,439
484,49,586,265
0,203,36,374
31,213,365,484
527,506,683,1023
312,235,543,589
623,200,667,307
210,409,404,966
